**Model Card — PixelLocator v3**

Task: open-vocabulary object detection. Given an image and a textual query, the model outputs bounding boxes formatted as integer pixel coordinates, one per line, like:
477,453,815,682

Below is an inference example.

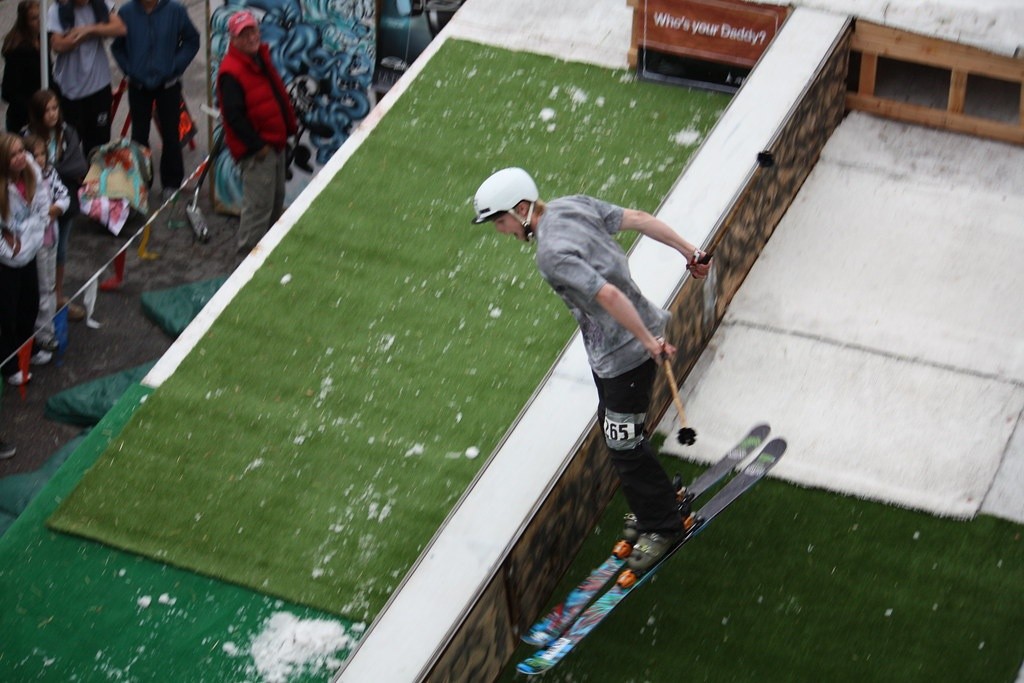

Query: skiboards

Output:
508,424,789,676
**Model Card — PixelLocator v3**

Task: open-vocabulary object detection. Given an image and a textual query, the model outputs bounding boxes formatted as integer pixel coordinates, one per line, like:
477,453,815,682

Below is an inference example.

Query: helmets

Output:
472,167,538,226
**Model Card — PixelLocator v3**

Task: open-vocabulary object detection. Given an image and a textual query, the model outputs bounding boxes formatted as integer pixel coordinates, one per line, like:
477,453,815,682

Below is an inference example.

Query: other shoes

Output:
29,348,52,365
35,332,60,348
0,442,17,459
7,371,32,386
162,184,177,209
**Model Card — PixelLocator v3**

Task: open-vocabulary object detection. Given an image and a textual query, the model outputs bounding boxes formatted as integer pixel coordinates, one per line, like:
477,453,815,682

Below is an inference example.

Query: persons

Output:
218,14,300,268
471,166,713,570
0,91,85,383
0,0,202,198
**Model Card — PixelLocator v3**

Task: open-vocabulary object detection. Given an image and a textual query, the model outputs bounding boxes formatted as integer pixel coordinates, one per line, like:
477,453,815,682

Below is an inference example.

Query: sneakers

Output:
624,512,641,543
628,523,685,571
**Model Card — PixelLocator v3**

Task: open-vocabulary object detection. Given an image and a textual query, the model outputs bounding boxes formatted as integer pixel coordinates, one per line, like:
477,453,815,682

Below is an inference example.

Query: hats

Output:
229,12,257,36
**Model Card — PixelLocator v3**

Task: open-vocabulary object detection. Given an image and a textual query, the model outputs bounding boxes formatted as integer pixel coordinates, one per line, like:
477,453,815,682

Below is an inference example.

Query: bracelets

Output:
687,248,701,270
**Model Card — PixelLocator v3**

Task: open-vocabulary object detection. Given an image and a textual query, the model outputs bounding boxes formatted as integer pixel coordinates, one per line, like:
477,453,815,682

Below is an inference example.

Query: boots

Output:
56,266,85,319
